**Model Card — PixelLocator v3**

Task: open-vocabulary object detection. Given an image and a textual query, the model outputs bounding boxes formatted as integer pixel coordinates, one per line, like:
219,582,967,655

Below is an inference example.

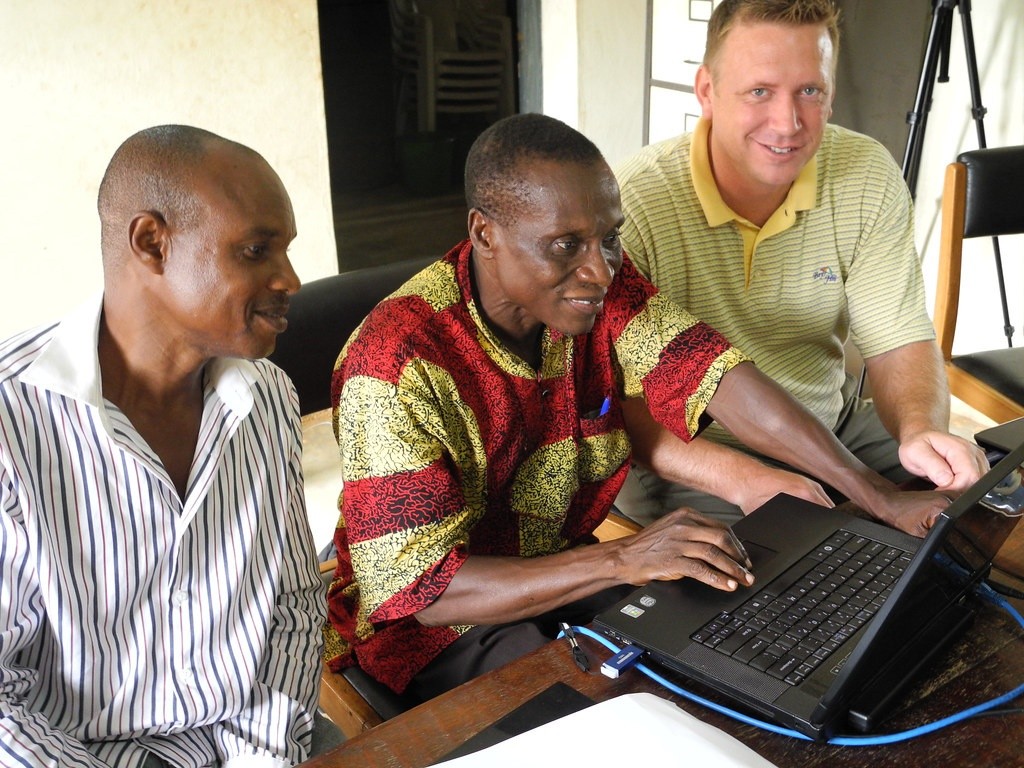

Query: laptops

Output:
590,443,1024,748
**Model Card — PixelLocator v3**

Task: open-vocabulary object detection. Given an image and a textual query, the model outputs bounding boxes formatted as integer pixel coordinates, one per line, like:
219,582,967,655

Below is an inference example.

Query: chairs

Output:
266,254,441,739
933,145,1024,425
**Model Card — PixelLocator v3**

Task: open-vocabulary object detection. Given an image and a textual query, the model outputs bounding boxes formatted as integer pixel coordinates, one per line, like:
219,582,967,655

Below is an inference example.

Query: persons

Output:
322,112,960,705
599,0,990,527
0,124,326,768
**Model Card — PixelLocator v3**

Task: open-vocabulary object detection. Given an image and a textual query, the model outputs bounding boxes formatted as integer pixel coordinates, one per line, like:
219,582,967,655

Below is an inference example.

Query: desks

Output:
294,478,1024,768
